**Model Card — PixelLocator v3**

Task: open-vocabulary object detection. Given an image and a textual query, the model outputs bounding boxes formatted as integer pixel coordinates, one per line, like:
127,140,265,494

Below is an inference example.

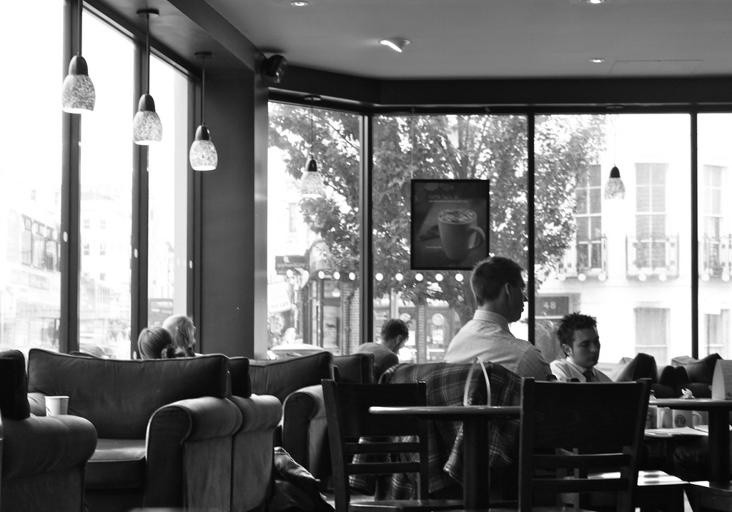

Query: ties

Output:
583,371,594,382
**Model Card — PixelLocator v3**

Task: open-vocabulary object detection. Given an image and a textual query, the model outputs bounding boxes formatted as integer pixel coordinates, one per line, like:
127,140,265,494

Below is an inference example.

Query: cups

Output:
671,407,705,430
435,207,486,263
656,406,673,429
43,393,72,417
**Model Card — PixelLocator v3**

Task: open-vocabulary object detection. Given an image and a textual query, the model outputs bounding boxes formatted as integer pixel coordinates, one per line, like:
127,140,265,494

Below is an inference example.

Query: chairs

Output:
495,372,654,512
546,372,689,512
319,377,464,511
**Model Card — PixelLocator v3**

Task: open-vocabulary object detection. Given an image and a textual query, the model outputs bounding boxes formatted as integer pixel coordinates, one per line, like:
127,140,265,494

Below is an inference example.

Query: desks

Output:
647,395,731,512
364,399,523,511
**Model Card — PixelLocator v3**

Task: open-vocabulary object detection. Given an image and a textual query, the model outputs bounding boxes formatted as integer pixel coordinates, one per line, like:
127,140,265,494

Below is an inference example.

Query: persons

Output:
135,324,178,363
348,319,408,386
440,256,551,390
161,313,197,357
547,312,621,388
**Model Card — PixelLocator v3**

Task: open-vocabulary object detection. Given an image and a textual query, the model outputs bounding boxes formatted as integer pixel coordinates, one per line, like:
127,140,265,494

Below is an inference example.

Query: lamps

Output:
300,91,327,200
59,0,100,116
604,79,627,201
126,4,169,149
187,45,221,174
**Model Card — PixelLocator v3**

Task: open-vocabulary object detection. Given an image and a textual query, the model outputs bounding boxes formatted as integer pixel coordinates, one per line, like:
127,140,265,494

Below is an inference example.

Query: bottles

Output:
646,388,660,431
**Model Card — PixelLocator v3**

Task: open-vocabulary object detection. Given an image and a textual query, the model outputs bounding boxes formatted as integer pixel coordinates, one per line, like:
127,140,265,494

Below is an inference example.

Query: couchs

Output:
332,351,375,501
26,349,244,512
192,351,337,479
69,350,283,512
609,349,731,400
0,347,99,512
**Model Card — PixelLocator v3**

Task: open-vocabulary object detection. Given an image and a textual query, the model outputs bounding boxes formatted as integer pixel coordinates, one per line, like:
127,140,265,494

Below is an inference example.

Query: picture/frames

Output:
408,176,493,274
